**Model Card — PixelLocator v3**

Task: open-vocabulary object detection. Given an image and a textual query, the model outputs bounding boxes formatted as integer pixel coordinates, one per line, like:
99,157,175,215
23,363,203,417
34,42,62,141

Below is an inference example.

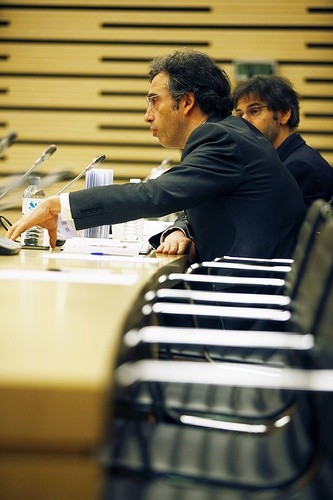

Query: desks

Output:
0,234,188,500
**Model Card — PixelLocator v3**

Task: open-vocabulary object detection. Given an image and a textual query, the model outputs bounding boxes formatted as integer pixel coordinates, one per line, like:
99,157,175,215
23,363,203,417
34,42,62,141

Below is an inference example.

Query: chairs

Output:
100,197,333,500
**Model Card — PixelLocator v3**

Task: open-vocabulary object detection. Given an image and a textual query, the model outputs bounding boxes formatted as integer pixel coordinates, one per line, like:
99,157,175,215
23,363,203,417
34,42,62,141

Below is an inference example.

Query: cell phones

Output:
20,245,51,250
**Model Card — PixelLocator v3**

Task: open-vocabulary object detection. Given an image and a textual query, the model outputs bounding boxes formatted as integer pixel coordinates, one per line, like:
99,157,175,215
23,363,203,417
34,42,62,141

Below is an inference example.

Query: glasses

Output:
235,106,269,118
146,91,187,107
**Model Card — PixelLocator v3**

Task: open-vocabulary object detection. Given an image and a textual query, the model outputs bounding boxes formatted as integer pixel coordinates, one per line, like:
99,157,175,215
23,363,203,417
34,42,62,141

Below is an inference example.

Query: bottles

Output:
20,176,45,248
123,178,143,243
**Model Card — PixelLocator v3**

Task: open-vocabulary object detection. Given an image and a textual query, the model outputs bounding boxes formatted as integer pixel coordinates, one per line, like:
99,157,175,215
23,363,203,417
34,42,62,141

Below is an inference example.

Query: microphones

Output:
56,154,106,197
1,144,57,199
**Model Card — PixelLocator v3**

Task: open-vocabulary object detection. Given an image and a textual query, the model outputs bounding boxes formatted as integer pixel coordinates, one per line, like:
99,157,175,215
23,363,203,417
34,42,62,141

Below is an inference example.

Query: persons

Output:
6,45,308,329
232,73,333,210
